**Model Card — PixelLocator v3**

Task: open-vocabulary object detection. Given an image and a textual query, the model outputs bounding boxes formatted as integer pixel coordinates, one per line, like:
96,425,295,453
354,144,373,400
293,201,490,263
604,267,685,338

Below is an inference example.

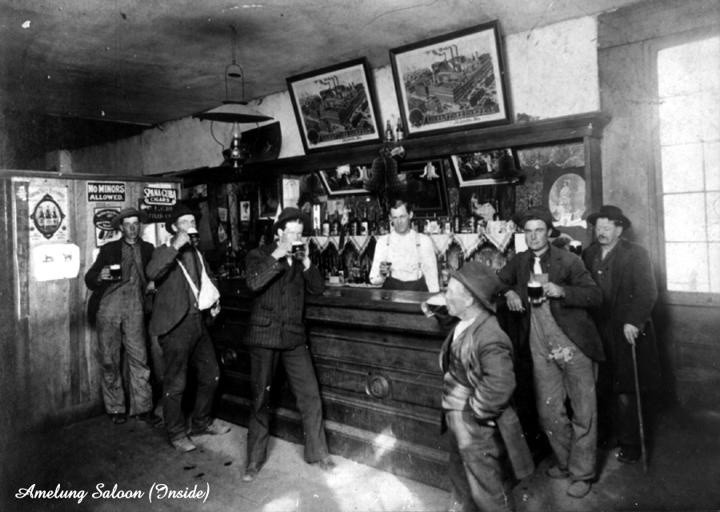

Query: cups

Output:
103,263,122,280
185,227,202,247
288,235,306,259
379,253,391,276
528,278,549,305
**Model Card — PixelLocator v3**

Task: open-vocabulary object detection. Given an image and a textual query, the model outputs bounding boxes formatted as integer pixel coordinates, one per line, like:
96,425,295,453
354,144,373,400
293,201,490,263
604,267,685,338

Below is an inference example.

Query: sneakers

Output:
243,463,262,481
617,447,643,464
317,456,335,470
138,413,162,425
547,465,571,478
567,480,591,497
168,433,196,451
191,421,230,435
109,414,127,424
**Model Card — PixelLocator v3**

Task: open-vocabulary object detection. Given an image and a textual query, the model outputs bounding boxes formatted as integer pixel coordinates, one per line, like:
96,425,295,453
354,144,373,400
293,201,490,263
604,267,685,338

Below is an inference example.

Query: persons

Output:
85,207,156,427
146,198,233,454
502,206,605,499
243,207,336,484
366,199,442,293
419,261,539,512
581,205,658,466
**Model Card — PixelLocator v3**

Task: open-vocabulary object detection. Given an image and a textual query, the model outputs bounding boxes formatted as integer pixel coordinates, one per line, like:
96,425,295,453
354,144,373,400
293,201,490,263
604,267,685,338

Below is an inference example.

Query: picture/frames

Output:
447,146,523,190
389,18,512,140
541,165,586,233
396,158,451,220
285,55,384,155
316,166,372,200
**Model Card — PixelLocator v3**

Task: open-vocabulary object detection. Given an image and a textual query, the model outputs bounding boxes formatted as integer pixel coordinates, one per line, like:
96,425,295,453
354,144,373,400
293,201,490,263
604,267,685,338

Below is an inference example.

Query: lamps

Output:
191,62,275,170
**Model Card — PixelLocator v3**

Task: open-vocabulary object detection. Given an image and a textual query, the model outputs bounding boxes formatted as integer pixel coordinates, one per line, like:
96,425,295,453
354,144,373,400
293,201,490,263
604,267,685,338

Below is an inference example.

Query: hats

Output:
165,200,201,233
110,207,146,230
449,260,501,314
273,207,309,234
586,205,631,231
512,206,561,237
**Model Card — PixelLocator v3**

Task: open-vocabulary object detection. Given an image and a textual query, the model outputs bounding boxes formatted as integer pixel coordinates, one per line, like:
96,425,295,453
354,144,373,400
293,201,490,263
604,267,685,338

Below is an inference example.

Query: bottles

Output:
310,253,372,285
320,197,499,235
